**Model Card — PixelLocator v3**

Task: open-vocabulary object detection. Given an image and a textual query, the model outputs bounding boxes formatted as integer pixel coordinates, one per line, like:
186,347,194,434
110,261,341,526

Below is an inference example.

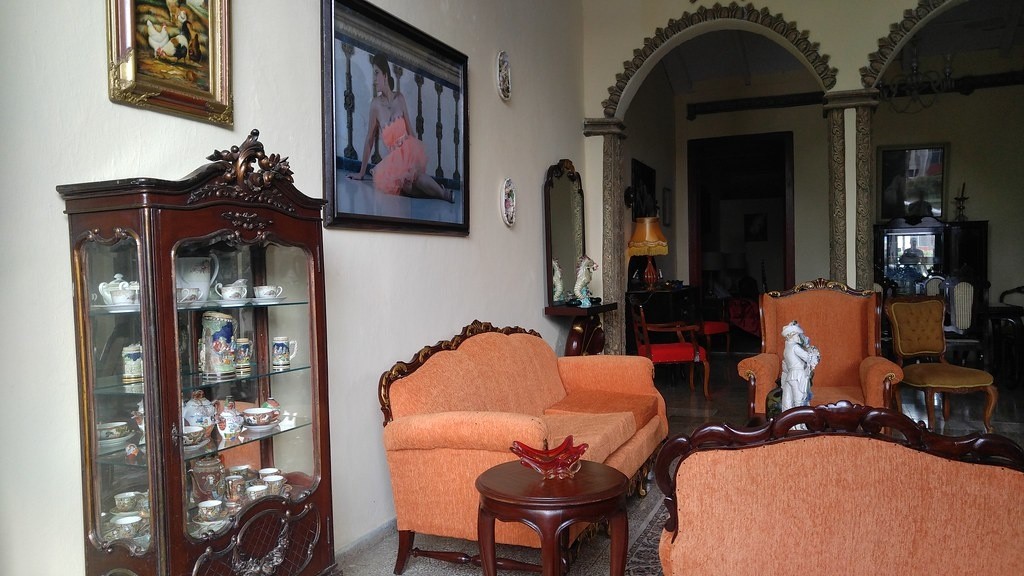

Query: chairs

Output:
630,297,731,402
737,277,903,440
883,293,995,436
879,270,1024,387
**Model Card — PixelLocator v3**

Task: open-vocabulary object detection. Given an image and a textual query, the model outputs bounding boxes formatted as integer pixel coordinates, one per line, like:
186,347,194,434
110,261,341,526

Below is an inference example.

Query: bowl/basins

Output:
182,426,204,445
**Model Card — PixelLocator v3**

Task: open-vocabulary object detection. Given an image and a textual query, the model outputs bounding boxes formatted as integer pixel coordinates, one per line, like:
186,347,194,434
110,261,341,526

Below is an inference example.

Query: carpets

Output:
623,495,670,576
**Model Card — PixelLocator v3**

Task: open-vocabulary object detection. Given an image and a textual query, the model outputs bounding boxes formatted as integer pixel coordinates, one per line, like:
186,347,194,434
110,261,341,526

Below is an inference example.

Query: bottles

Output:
261,398,281,423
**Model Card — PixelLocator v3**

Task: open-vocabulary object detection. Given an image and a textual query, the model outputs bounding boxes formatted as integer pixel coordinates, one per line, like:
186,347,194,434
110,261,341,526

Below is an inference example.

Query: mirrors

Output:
541,159,584,308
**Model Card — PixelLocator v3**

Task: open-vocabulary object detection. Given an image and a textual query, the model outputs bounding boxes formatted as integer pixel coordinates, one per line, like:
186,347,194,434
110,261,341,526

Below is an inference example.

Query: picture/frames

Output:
106,0,235,134
743,213,768,242
662,187,673,226
874,142,952,225
320,0,470,238
630,157,656,222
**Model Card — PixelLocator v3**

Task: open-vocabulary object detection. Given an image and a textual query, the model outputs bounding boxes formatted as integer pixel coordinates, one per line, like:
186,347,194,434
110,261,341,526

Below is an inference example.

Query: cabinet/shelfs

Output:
874,219,989,351
625,285,698,387
54,128,344,576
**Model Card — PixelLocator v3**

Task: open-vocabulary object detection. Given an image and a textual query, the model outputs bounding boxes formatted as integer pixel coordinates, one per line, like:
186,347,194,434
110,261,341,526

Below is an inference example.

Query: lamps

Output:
887,40,976,114
628,217,669,291
701,252,725,297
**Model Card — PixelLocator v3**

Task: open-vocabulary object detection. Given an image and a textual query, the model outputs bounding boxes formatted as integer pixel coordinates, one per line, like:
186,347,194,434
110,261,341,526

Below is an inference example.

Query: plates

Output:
98,433,135,447
213,299,250,307
250,297,287,305
177,301,209,308
109,507,139,515
95,305,141,313
244,425,276,430
192,515,228,525
183,437,210,452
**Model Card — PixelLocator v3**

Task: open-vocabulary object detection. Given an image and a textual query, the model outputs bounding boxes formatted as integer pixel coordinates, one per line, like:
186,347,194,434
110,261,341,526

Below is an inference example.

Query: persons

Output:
347,53,456,204
781,320,820,430
905,237,928,275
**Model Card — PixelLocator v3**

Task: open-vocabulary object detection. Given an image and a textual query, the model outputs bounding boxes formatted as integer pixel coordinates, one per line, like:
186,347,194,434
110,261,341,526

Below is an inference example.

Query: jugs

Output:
188,455,249,514
184,390,244,439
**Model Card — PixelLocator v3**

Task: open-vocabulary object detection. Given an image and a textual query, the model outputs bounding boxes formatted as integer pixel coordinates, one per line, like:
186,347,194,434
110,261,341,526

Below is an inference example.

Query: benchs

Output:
652,400,1024,576
377,319,668,576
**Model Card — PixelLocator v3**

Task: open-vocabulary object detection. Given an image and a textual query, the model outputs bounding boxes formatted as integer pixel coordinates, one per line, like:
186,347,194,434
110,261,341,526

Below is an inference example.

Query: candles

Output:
960,182,966,197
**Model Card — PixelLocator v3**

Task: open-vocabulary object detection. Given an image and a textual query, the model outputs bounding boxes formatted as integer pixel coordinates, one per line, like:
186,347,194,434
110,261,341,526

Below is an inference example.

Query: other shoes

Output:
441,184,454,203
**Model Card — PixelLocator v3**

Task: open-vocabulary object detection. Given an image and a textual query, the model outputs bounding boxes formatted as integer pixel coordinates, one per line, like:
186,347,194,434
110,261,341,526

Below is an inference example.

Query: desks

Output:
475,459,630,576
987,301,1024,391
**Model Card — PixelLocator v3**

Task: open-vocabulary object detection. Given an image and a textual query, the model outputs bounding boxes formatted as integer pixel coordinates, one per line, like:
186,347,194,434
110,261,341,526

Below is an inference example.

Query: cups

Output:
122,343,142,384
263,475,284,496
247,484,267,502
99,273,139,303
259,467,281,478
198,311,253,382
97,421,127,439
253,286,283,297
244,407,280,424
198,500,222,521
114,492,137,511
116,516,142,537
272,337,298,371
175,252,219,308
215,282,247,299
